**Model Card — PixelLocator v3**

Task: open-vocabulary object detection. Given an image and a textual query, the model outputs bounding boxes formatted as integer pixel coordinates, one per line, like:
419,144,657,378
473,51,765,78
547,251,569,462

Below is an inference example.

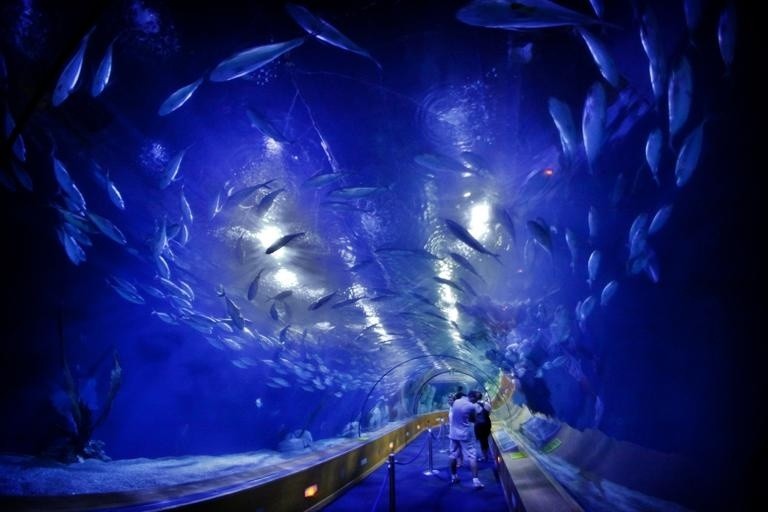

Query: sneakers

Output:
473,481,485,489
452,476,460,484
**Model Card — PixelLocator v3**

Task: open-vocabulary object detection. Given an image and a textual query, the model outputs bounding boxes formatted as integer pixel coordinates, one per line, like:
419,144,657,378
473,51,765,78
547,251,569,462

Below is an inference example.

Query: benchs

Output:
491,428,574,511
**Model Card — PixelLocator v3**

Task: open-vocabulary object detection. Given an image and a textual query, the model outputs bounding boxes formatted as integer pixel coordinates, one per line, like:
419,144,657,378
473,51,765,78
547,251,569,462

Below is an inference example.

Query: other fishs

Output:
1,2,736,395
255,398,262,408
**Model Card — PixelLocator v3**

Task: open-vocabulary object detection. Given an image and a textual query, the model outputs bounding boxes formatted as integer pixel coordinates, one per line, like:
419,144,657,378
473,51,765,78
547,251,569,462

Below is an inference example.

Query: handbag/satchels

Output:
474,414,491,440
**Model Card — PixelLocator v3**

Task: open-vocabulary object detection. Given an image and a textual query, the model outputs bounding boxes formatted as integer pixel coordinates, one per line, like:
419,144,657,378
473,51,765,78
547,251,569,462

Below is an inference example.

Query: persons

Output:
447,390,485,487
448,386,491,467
